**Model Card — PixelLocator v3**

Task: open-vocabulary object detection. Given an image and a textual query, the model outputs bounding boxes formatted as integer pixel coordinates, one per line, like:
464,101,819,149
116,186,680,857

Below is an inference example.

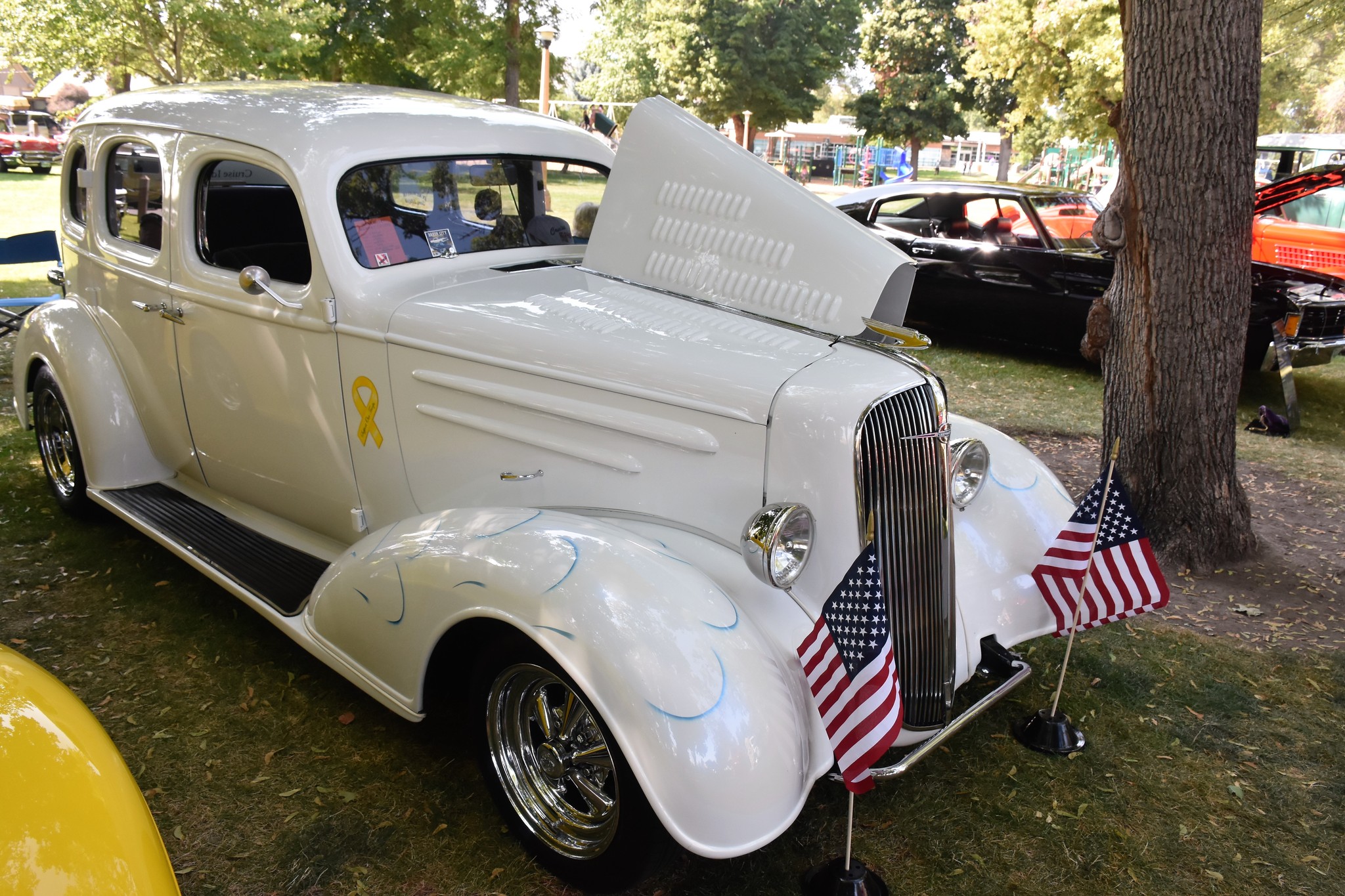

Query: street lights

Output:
534,22,559,211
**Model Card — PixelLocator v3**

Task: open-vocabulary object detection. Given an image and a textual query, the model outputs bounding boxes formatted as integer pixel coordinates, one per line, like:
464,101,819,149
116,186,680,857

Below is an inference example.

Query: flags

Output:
1032,438,1170,639
797,508,907,795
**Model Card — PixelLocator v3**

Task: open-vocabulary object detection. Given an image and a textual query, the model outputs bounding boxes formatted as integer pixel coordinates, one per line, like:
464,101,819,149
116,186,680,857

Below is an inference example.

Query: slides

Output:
1065,155,1105,187
883,147,915,185
878,168,890,181
1017,163,1041,184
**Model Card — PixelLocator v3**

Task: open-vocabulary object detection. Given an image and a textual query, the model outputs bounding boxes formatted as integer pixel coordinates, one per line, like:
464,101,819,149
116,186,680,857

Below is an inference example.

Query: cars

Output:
825,178,1345,379
8,75,1080,896
1252,132,1345,280
0,109,67,174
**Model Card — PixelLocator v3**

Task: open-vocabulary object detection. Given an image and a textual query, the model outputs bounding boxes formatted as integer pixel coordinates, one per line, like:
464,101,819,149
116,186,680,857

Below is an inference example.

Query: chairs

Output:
980,217,1024,245
936,218,977,241
0,231,66,337
